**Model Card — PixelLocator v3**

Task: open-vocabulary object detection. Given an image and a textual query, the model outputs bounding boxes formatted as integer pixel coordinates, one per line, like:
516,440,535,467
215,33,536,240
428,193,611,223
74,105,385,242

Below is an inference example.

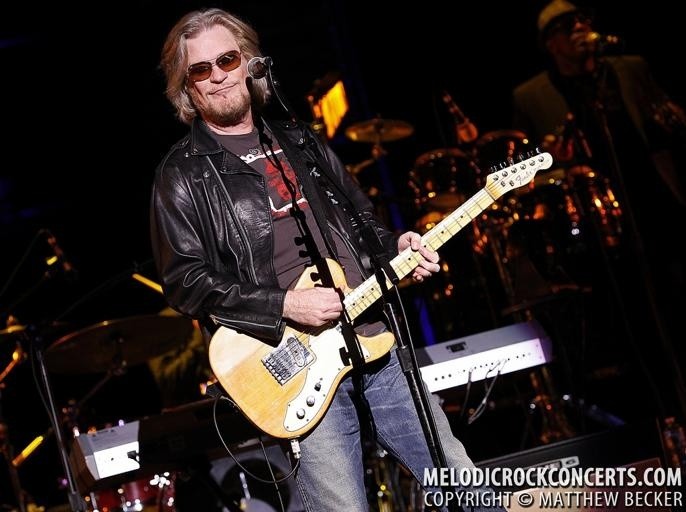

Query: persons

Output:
145,305,222,408
148,8,508,512
509,0,686,258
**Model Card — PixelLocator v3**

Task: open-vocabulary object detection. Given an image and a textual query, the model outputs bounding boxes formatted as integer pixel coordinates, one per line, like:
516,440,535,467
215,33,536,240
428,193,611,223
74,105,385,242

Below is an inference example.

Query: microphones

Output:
442,94,478,142
588,32,621,45
47,236,71,272
247,57,273,79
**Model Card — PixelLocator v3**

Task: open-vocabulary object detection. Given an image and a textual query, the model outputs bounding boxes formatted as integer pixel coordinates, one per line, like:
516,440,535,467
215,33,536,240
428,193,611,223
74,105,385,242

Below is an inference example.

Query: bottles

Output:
442,94,489,145
663,416,686,471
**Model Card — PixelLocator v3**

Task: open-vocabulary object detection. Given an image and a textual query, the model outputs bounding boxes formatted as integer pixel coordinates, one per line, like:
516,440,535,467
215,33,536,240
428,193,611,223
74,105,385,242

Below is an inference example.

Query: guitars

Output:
207,146,553,440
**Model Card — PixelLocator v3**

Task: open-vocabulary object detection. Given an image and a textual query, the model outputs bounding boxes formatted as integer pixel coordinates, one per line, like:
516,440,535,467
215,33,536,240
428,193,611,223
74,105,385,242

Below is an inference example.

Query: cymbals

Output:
346,117,412,143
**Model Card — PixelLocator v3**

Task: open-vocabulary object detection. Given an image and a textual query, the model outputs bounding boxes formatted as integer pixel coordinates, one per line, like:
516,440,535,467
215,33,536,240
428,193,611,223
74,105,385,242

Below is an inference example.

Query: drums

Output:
477,129,533,182
414,149,472,211
519,162,628,269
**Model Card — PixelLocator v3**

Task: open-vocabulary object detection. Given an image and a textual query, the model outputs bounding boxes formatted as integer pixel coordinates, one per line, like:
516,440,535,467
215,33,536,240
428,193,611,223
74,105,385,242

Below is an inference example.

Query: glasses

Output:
184,45,243,83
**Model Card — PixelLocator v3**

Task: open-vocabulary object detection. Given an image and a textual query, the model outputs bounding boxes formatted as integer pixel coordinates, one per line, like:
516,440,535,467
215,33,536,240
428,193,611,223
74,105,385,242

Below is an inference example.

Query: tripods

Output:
471,225,632,452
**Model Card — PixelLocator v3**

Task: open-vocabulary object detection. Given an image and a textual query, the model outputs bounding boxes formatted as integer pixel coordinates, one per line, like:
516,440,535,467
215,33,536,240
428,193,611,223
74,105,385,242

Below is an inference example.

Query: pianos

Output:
68,319,547,495
